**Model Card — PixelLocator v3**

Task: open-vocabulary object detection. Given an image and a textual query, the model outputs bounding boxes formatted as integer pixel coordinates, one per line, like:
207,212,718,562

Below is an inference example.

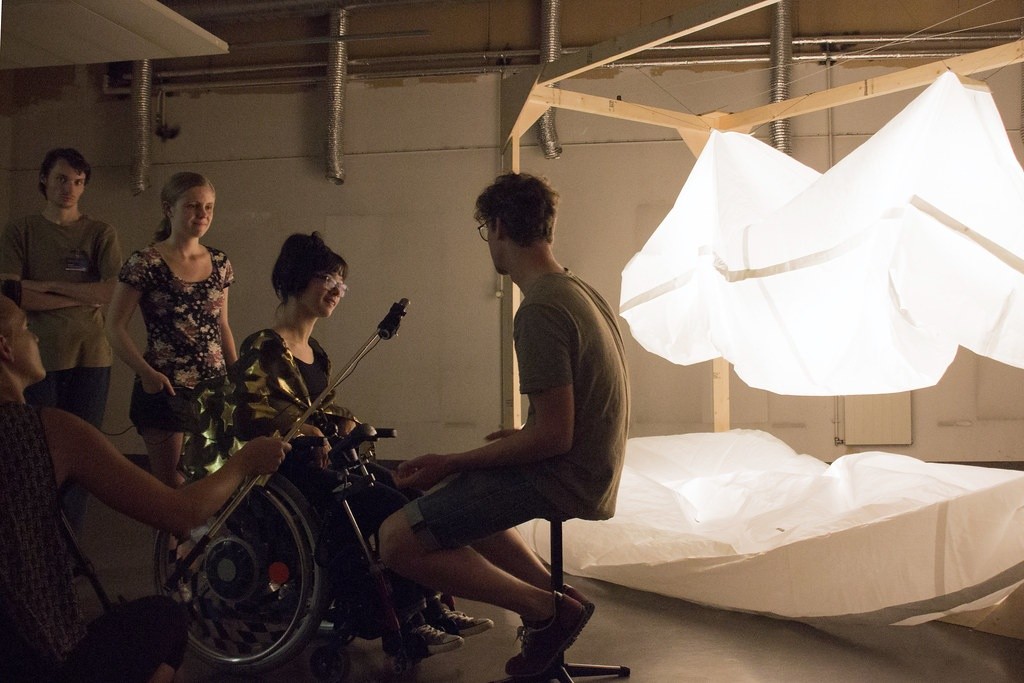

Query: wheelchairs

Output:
153,417,456,683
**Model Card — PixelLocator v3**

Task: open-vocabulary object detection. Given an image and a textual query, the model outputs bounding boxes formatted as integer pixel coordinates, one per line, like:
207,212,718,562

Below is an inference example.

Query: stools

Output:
489,522,630,683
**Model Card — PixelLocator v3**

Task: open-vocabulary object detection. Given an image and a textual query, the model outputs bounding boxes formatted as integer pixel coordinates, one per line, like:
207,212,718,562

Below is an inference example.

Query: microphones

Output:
377,298,411,340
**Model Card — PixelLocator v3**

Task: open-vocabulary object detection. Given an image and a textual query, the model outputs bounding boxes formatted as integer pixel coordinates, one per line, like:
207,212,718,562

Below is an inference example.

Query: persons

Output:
0,147,125,529
0,291,293,683
379,168,629,683
105,171,240,603
235,230,495,656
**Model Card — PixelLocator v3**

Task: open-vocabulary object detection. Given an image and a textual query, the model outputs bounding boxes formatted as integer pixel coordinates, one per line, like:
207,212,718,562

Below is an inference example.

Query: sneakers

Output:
506,584,595,675
382,604,494,655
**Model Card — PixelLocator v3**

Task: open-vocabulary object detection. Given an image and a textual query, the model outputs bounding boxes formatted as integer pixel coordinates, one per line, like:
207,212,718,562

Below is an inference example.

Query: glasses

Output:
316,272,347,297
478,218,496,241
